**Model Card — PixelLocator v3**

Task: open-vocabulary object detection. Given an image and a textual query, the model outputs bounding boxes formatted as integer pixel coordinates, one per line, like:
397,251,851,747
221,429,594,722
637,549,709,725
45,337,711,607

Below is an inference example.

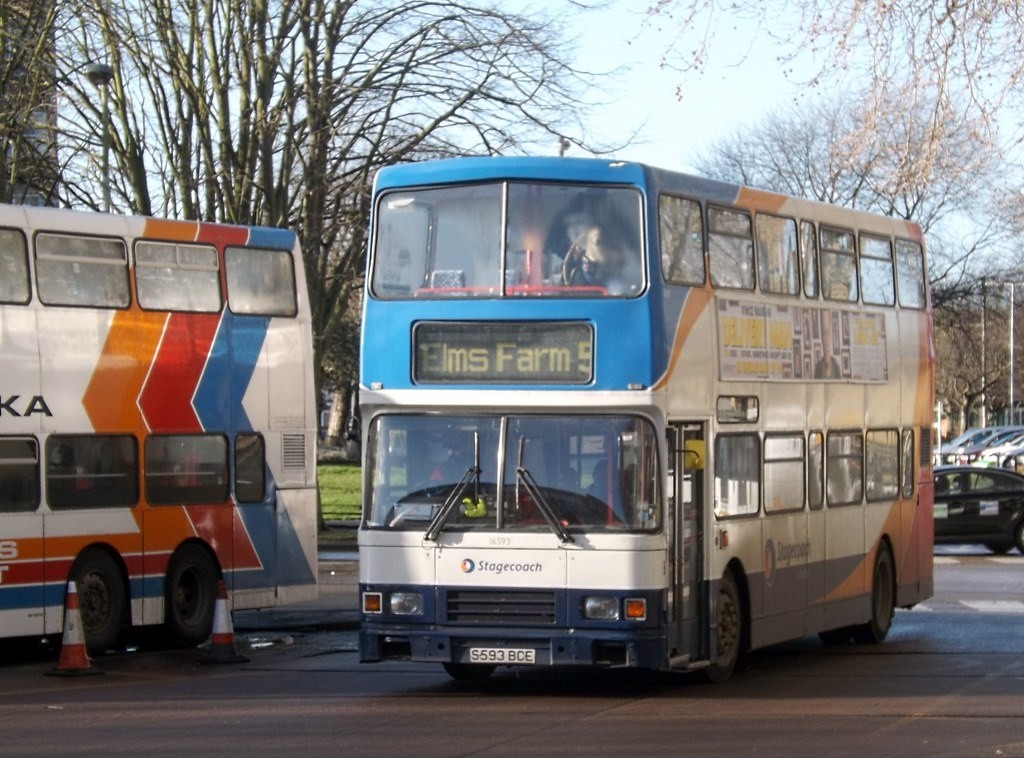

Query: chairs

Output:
431,270,468,297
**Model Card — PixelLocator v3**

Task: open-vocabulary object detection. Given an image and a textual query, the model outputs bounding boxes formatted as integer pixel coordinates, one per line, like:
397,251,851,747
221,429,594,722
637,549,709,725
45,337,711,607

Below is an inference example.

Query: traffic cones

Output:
195,579,251,664
44,579,105,678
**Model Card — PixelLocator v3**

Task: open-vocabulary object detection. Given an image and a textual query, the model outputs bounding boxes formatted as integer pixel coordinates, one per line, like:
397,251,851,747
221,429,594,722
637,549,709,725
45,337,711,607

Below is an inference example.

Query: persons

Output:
583,458,634,525
431,433,476,483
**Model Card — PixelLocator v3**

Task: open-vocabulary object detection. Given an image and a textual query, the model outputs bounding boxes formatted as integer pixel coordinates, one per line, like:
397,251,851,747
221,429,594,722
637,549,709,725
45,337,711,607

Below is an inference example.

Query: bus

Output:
0,202,323,663
323,155,935,689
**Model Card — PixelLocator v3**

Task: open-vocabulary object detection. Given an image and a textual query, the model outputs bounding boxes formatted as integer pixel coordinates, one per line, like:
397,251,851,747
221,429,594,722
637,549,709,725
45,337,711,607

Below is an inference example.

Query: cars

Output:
924,423,1024,557
